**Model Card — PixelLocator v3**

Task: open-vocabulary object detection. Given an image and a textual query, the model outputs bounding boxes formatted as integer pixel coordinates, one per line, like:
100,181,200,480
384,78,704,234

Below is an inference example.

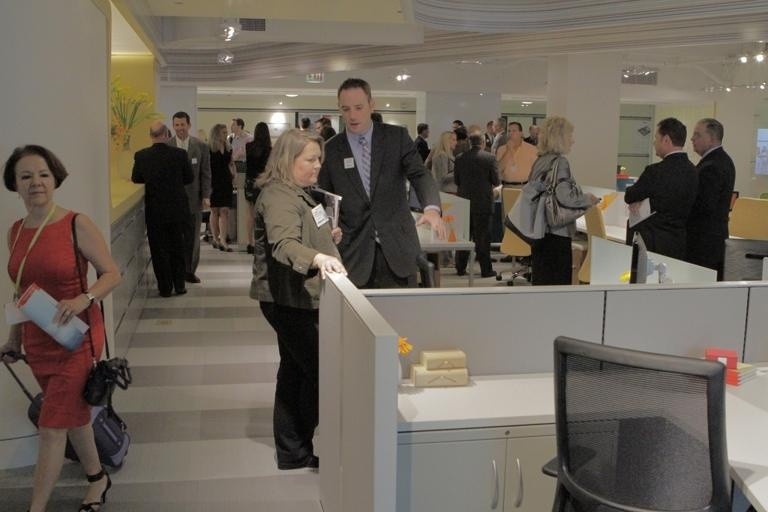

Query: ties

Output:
358,136,375,202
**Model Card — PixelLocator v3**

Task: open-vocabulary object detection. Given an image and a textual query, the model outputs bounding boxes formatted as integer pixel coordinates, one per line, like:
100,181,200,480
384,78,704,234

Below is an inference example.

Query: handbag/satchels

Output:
544,155,589,229
81,356,132,407
502,178,550,254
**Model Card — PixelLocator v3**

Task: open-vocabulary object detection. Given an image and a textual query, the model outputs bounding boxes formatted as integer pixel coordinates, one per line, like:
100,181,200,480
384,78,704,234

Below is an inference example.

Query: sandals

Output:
211,239,234,253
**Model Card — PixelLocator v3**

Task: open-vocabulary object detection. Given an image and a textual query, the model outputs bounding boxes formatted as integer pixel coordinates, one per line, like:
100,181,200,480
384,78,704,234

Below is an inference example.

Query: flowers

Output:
109,72,167,149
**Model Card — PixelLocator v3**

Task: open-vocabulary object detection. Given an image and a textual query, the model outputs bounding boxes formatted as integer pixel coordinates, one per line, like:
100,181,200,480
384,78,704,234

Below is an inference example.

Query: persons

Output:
371,113,598,286
625,118,699,283
317,79,446,289
131,111,273,297
685,118,736,280
0,144,122,512
297,117,337,142
249,130,349,470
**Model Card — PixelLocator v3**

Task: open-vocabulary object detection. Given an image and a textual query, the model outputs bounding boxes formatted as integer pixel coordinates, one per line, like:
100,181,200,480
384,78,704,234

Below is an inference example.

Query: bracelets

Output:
82,290,95,305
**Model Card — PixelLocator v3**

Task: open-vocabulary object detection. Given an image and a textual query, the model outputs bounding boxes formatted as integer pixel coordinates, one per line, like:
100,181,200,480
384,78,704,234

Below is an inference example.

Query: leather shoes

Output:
277,452,319,469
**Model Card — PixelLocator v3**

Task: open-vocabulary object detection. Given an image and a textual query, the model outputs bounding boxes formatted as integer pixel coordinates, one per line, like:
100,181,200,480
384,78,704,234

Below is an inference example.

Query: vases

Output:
116,144,135,181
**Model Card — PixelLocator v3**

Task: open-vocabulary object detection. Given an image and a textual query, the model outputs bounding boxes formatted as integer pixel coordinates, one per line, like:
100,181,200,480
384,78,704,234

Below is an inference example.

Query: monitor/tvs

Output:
409,183,424,214
629,229,649,285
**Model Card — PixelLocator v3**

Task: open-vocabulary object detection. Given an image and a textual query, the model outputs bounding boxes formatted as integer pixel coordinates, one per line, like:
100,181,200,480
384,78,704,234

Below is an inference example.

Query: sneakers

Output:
160,271,204,298
457,267,498,278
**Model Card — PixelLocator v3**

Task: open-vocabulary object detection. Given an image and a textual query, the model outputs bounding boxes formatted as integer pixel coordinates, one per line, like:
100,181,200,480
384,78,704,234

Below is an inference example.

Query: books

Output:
311,189,343,230
700,358,757,386
16,283,90,352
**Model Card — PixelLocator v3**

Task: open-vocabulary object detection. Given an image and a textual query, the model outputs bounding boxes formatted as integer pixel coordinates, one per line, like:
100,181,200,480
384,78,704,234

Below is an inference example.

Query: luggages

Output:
27,391,131,470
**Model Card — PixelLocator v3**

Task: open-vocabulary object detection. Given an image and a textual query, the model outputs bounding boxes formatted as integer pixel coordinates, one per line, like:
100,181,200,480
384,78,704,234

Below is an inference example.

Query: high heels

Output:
78,464,111,512
